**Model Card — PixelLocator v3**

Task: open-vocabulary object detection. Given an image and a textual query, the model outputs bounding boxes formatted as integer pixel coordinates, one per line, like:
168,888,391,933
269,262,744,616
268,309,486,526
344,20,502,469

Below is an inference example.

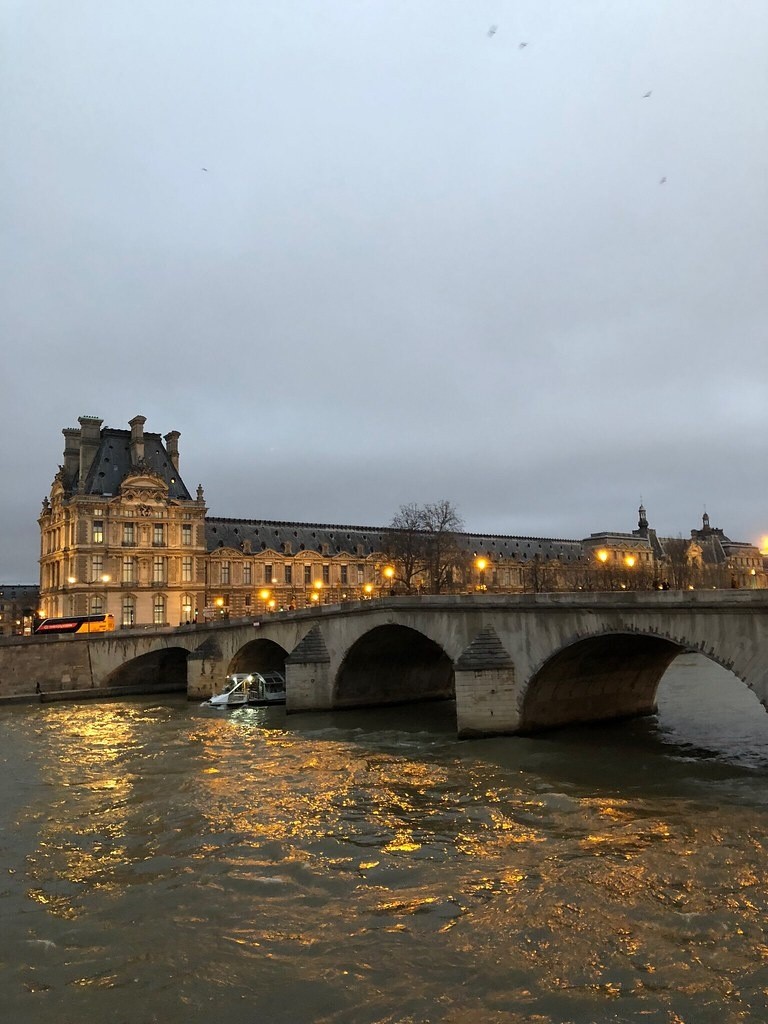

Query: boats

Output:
207,670,286,706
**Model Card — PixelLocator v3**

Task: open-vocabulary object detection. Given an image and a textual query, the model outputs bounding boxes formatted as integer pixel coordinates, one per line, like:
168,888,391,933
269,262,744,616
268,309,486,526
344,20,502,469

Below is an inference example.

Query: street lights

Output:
752,568,756,589
477,559,486,594
626,557,636,591
386,568,393,597
316,581,322,606
599,551,608,592
261,591,269,614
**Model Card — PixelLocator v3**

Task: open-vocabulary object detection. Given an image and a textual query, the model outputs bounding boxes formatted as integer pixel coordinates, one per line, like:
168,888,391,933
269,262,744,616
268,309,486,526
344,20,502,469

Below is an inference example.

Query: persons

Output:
651,577,670,591
731,573,738,589
289,605,294,610
35,682,41,694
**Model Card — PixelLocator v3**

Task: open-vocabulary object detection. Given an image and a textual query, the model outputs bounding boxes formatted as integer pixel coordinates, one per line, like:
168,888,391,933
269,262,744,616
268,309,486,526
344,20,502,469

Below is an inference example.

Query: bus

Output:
34,613,115,632
